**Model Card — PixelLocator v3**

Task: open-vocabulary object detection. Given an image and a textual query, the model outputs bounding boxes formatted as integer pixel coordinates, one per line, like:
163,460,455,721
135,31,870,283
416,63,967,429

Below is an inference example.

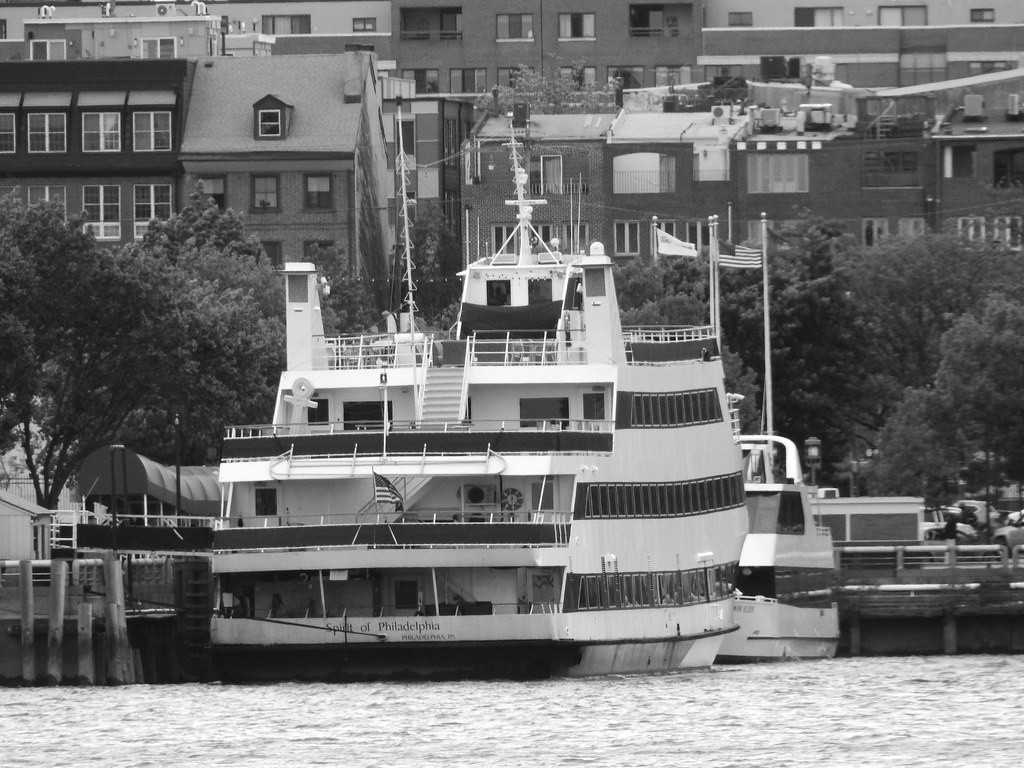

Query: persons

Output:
702,348,710,362
943,518,957,566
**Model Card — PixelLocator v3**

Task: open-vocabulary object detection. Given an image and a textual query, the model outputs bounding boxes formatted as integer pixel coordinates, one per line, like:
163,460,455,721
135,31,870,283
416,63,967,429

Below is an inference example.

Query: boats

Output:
206,94,750,681
695,210,840,664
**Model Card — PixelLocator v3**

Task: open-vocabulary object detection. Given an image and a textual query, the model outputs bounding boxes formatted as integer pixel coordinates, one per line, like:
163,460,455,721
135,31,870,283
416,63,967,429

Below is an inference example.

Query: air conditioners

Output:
155,3,176,17
711,105,731,119
464,485,494,506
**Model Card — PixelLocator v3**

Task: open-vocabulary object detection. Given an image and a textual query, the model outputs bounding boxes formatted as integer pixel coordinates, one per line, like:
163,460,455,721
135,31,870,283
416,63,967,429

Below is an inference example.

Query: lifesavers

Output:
502,488,522,510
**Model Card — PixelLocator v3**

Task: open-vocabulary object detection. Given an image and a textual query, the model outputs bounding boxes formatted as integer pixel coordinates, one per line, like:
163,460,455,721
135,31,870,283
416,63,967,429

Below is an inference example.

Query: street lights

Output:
804,435,821,499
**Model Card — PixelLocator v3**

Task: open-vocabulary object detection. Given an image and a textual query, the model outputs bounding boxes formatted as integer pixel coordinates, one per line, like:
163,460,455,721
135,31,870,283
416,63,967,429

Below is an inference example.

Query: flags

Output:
373,473,404,512
717,238,762,269
656,228,698,257
